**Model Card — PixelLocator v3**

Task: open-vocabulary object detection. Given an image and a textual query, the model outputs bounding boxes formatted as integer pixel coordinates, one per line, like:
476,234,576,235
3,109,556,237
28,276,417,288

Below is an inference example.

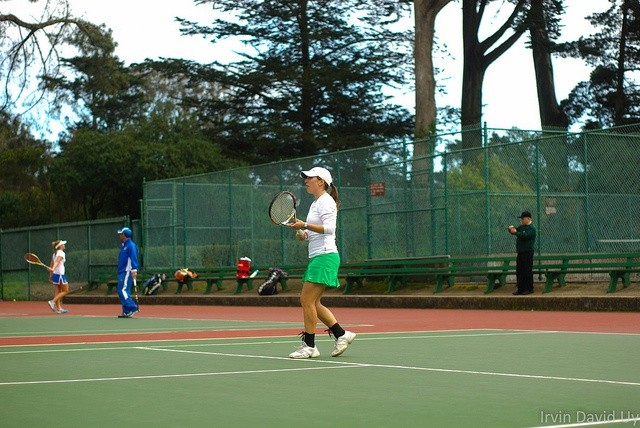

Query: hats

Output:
117,227,133,237
301,167,333,187
517,211,531,218
55,240,67,249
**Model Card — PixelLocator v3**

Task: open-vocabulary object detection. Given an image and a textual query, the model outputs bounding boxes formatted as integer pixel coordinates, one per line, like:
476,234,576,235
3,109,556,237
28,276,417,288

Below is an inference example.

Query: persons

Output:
270,167,356,359
116,227,139,318
508,212,536,295
50,239,69,313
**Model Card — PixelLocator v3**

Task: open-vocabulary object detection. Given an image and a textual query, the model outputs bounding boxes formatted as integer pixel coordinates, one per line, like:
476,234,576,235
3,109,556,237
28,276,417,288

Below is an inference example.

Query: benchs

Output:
88,252,640,294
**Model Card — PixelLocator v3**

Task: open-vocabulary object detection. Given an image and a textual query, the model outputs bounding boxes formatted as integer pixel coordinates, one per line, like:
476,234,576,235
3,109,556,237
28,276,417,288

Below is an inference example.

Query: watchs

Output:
301,226,307,231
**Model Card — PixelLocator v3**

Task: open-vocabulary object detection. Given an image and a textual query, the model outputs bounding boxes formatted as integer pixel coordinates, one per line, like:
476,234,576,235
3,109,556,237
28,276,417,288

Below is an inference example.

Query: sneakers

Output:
127,309,139,317
48,300,56,312
118,313,132,318
331,330,357,357
289,342,320,359
56,308,69,314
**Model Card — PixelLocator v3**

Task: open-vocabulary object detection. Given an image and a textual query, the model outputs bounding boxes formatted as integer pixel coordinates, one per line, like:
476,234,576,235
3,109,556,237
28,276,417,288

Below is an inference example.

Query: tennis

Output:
13,299,16,302
